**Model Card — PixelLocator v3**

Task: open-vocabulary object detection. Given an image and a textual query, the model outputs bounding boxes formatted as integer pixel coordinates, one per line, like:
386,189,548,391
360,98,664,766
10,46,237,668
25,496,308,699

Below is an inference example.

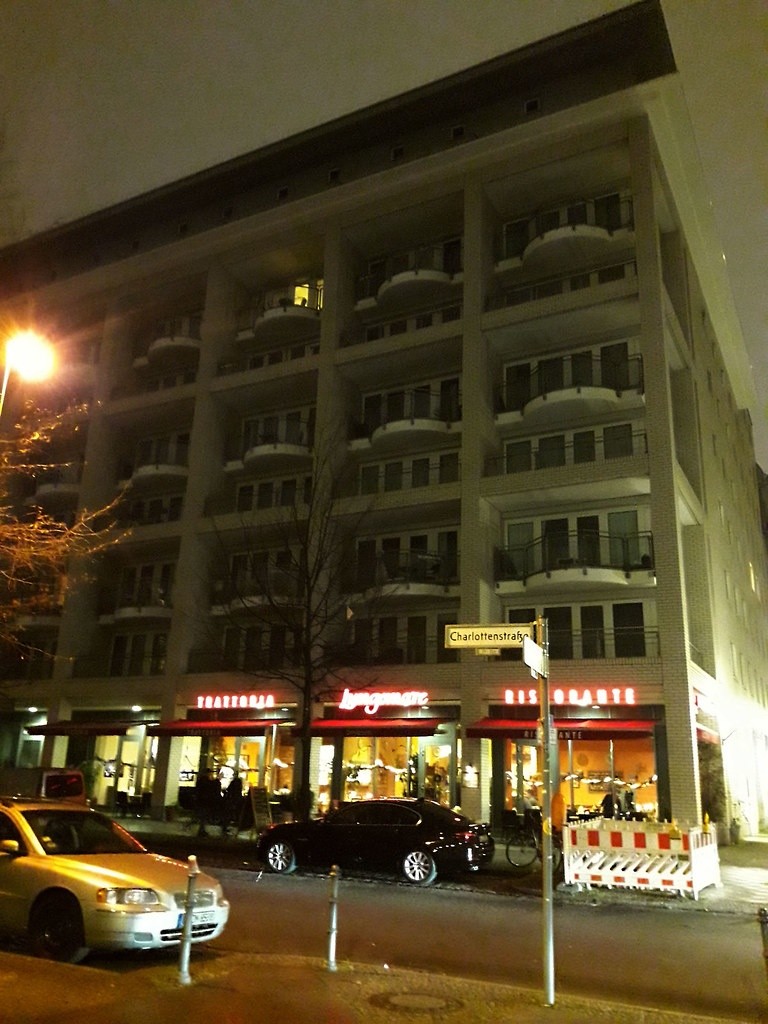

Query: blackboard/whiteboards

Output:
249,786,272,822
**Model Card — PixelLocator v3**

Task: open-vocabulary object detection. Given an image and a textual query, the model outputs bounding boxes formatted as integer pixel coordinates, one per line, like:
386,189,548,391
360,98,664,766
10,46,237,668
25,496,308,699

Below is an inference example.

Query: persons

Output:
624,783,634,811
227,771,242,826
596,787,622,818
214,771,230,832
196,768,214,837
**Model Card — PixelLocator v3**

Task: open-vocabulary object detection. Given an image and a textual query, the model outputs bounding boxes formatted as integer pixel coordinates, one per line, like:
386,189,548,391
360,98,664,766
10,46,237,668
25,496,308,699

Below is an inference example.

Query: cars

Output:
0,795,231,968
256,796,497,888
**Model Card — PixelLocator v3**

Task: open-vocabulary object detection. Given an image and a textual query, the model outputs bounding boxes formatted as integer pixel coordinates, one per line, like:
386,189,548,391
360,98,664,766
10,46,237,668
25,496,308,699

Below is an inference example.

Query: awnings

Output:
146,718,295,736
292,716,452,737
23,720,161,736
465,716,655,740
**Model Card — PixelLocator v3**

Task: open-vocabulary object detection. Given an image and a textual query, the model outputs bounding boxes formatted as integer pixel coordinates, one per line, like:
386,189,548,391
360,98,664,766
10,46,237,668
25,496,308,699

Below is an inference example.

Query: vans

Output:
0,764,87,826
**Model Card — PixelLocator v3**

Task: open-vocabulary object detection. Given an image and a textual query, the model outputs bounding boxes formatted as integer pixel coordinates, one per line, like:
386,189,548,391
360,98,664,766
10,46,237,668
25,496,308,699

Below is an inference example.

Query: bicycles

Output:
505,805,569,877
